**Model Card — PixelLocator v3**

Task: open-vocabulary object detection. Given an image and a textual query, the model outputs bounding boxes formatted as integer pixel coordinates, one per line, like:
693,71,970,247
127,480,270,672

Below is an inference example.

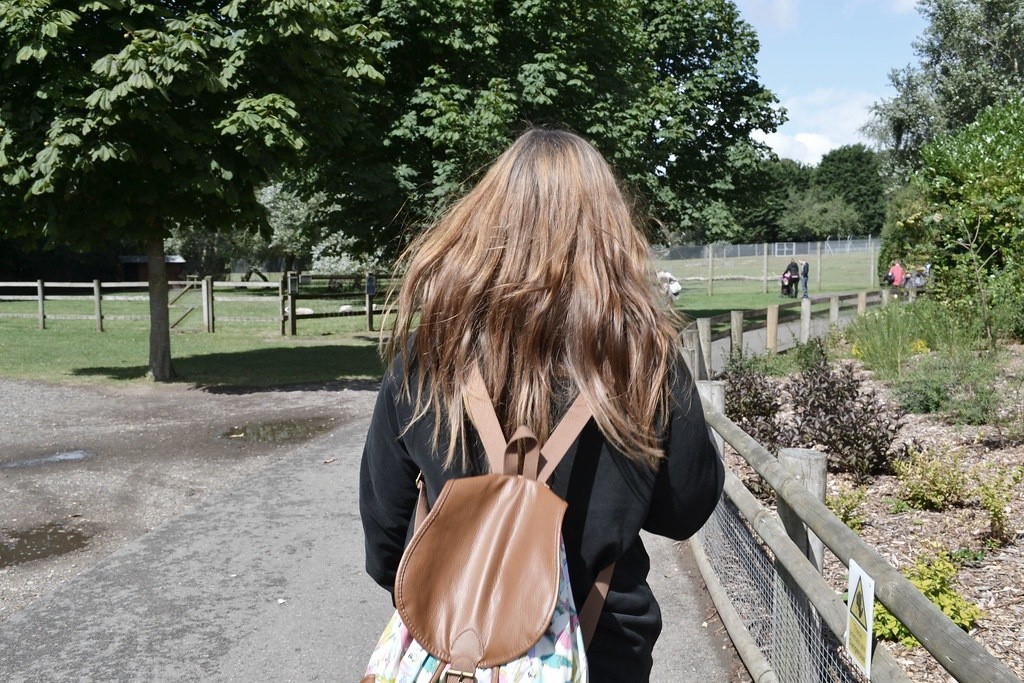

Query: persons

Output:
358,129,725,683
888,258,906,288
782,258,800,298
799,258,809,298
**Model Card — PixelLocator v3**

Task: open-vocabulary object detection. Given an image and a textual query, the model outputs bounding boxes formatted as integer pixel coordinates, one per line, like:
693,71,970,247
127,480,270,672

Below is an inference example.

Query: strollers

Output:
778,273,795,297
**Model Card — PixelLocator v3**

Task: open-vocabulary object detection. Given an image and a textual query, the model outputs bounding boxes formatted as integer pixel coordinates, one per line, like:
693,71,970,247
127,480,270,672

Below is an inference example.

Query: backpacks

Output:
358,343,615,683
